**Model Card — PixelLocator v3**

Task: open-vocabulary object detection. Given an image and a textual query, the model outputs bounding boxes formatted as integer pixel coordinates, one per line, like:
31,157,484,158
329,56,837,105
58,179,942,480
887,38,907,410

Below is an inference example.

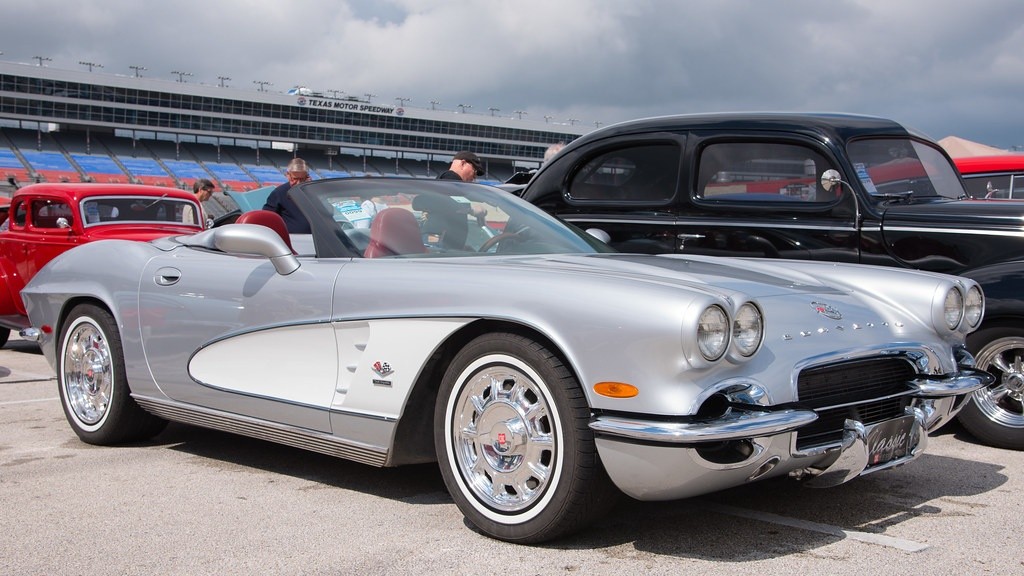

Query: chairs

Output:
236,210,298,255
364,208,433,258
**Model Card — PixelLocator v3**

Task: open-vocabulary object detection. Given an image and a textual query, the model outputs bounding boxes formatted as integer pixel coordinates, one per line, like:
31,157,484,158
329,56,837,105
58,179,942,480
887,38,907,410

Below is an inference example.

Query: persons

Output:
262,158,323,234
412,150,487,248
181,179,215,230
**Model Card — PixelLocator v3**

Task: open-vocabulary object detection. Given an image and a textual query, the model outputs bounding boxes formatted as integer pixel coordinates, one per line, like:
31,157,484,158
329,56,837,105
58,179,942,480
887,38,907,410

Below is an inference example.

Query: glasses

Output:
205,189,212,195
289,174,306,182
467,164,478,175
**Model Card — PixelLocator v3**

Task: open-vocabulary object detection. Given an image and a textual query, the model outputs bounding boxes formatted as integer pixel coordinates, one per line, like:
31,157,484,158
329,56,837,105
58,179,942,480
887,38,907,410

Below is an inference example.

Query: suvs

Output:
493,110,1024,454
0,184,211,349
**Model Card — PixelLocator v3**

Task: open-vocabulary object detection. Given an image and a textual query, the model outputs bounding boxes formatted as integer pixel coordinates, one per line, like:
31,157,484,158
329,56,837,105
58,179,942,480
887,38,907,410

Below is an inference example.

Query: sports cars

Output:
20,173,1000,546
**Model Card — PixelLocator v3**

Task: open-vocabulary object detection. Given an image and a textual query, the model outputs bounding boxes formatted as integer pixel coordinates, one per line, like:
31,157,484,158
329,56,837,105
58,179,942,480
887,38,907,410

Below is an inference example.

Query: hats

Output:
454,151,486,176
